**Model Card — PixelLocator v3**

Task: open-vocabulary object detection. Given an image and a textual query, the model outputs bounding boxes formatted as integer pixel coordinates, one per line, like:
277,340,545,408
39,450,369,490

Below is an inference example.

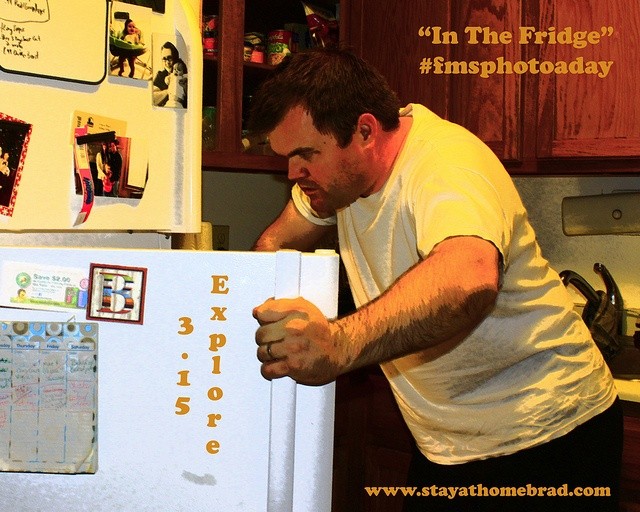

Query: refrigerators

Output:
0,0,340,511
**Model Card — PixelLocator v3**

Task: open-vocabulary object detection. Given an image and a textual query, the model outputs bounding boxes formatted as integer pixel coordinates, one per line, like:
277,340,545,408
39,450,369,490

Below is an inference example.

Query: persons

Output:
118,20,145,78
252,47,624,511
102,172,114,196
1,153,11,201
153,41,186,108
107,141,122,181
96,144,109,196
165,61,187,108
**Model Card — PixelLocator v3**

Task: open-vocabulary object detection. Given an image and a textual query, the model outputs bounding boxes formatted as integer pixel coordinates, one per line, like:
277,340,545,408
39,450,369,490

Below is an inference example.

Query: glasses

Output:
162,57,172,62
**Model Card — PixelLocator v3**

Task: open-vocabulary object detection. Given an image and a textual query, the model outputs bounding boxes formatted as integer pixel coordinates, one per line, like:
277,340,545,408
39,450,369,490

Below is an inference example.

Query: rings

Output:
267,342,275,361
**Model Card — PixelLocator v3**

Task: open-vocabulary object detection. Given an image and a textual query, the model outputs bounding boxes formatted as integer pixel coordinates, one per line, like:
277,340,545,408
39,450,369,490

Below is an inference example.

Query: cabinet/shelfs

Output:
205,1,350,176
348,2,521,177
521,1,638,176
363,381,404,512
332,377,369,512
